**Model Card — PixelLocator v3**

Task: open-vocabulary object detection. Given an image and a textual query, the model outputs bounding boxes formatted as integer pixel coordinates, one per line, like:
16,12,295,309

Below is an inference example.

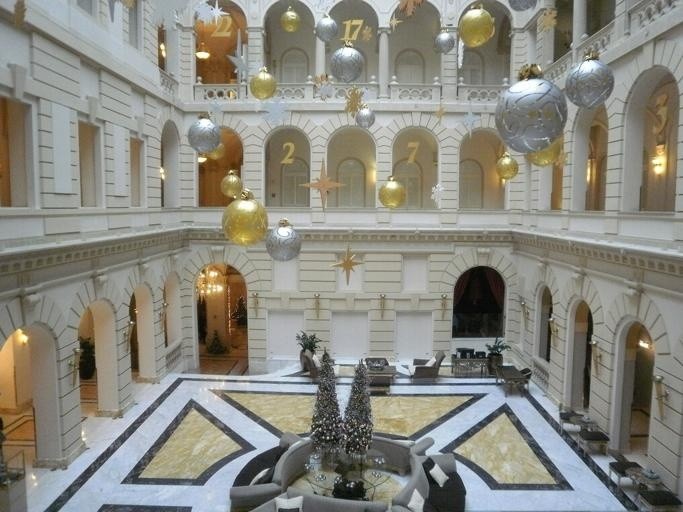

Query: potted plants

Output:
295,329,327,370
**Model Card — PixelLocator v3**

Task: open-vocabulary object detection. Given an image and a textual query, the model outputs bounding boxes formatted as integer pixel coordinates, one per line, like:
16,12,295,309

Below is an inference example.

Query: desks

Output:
579,421,598,431
636,490,683,511
576,430,609,458
607,461,643,492
496,365,527,399
557,413,584,435
450,356,490,378
626,468,660,489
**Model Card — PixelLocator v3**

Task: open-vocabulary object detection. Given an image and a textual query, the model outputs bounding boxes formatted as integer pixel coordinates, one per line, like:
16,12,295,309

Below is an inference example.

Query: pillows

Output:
423,503,435,512
406,488,423,512
420,456,436,482
248,465,271,485
254,466,274,484
429,464,449,487
273,495,303,511
381,433,407,439
277,507,299,511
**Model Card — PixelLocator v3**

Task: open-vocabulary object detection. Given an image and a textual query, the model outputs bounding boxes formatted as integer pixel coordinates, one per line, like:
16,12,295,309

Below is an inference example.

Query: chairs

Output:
359,374,395,396
302,348,339,384
511,368,531,393
407,349,445,380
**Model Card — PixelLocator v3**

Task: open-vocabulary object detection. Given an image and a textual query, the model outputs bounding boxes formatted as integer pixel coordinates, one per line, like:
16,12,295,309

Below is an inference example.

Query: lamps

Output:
192,15,214,64
195,267,222,303
651,373,670,420
158,302,169,329
440,294,447,321
548,317,559,347
122,321,135,353
67,347,83,388
252,293,259,316
519,301,529,326
379,294,385,317
313,293,319,315
588,340,601,374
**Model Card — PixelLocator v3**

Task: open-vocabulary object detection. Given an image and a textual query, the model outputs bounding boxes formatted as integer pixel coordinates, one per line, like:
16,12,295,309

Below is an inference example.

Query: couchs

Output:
229,431,312,506
388,451,468,512
359,431,434,476
246,487,389,511
